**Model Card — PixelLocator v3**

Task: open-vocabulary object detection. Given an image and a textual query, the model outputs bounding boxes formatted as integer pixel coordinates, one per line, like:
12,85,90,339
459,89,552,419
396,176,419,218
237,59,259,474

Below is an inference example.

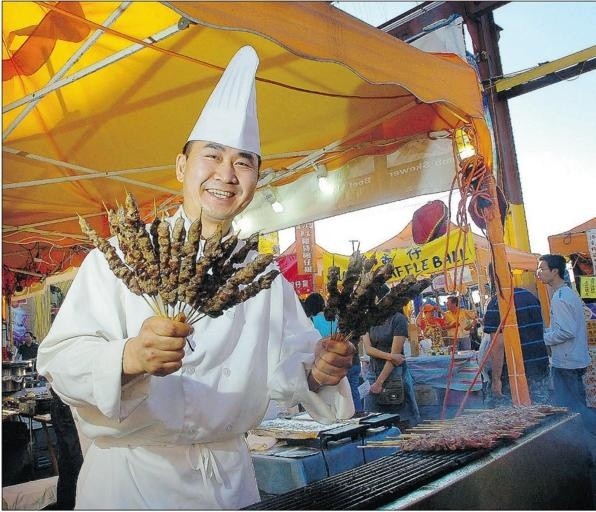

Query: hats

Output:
423,304,436,312
183,44,263,160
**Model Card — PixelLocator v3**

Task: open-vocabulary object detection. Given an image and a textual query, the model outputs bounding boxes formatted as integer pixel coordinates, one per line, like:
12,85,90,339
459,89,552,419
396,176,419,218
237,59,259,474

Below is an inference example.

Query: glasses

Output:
536,266,550,273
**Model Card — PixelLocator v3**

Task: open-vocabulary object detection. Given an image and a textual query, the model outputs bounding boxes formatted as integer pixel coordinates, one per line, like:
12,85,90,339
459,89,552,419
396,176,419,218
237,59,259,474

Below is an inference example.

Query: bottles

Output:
403,338,411,357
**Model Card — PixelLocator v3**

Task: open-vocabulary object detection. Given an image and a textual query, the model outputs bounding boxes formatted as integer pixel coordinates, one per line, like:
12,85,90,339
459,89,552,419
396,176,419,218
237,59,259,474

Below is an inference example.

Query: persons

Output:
37,44,358,510
303,293,491,432
13,332,39,388
486,261,550,405
537,255,595,464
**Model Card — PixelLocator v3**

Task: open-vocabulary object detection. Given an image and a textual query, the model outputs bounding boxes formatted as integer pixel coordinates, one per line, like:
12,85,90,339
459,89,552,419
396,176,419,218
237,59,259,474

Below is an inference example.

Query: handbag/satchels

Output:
375,380,405,406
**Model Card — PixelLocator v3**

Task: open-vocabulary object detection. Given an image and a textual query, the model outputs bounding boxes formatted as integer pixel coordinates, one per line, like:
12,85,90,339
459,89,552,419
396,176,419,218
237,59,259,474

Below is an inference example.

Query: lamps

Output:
233,153,332,227
